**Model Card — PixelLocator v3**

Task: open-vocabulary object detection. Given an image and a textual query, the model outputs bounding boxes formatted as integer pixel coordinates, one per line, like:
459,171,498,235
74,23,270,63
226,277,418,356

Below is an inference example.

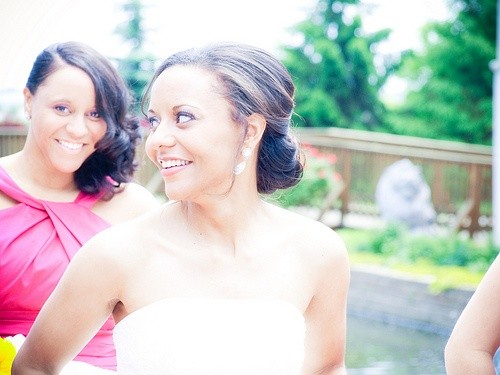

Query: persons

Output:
0,43,142,375
444,251,500,375
10,45,353,375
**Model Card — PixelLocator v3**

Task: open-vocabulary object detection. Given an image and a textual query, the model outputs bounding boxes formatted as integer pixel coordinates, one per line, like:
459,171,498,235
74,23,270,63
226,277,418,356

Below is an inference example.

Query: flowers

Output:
0,332,27,375
